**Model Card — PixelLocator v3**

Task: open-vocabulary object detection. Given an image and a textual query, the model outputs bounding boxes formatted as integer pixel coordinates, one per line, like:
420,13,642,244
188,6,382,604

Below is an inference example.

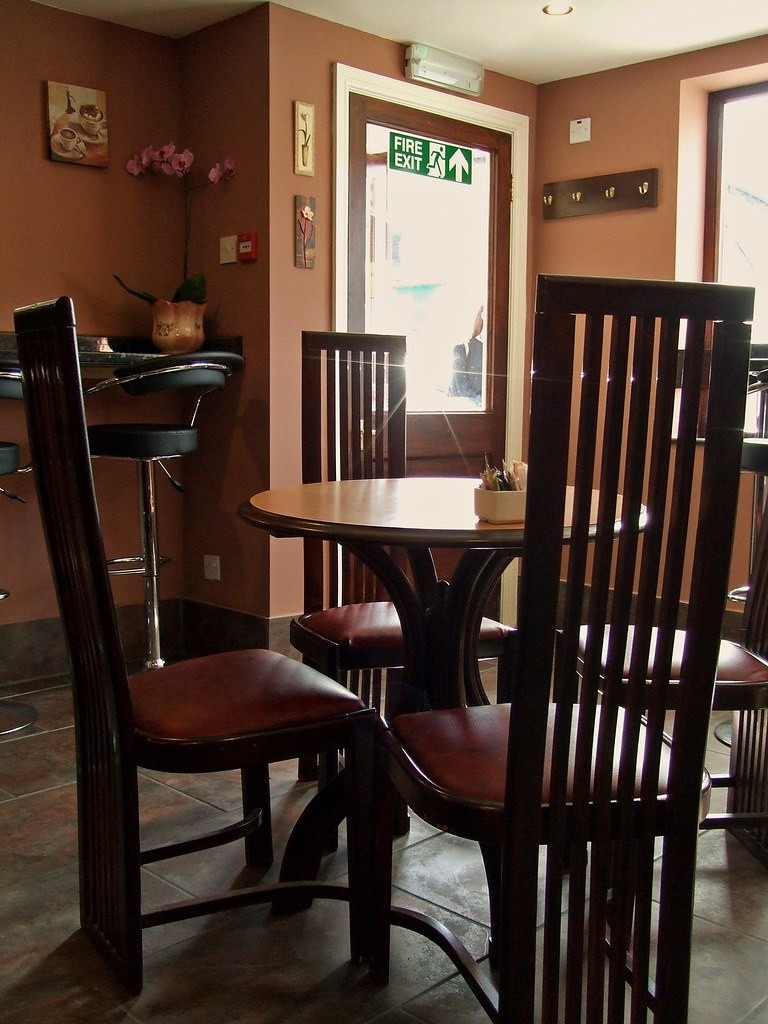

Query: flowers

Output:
113,141,238,305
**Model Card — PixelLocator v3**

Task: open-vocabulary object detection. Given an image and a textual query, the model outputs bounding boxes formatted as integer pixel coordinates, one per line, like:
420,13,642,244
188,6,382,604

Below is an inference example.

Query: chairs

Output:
301,329,520,857
554,624,768,950
378,276,757,1024
13,294,379,1001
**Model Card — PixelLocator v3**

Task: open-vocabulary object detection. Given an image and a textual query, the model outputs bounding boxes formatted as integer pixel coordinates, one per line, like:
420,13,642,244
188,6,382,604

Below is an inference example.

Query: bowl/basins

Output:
474,488,525,523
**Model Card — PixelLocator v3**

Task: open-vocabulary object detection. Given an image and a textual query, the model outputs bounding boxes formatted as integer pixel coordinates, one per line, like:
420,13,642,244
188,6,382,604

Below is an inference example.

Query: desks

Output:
236,475,655,1024
0,330,244,380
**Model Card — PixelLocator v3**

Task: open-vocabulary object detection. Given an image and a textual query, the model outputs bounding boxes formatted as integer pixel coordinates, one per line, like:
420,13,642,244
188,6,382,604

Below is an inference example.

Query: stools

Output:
83,363,233,673
0,370,40,735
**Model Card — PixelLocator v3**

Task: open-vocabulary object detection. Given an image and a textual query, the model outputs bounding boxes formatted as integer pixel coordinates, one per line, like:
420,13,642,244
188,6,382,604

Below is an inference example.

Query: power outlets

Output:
203,555,221,580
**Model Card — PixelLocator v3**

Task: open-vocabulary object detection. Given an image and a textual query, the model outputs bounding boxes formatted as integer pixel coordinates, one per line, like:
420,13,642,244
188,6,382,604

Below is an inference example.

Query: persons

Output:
448,303,488,397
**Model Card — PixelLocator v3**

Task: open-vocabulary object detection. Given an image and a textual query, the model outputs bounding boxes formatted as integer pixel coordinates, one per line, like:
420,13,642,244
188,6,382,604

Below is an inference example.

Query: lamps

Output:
405,42,485,97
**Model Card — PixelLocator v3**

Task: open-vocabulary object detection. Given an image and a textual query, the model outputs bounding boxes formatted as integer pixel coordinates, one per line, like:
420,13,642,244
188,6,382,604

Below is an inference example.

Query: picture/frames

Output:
294,100,316,177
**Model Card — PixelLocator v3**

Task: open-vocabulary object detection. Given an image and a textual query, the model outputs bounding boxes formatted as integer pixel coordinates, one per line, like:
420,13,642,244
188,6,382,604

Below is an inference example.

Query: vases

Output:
151,299,208,354
